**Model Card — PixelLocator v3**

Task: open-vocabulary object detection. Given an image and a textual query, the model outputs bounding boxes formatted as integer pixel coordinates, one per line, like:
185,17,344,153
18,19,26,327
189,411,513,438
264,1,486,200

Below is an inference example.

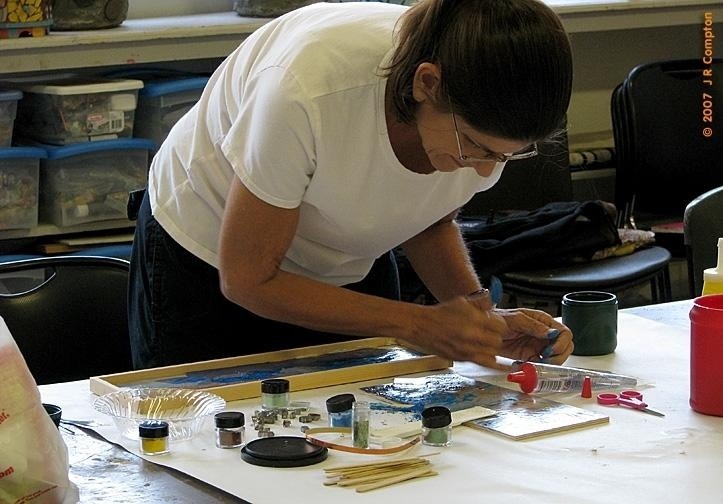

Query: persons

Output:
122,0,576,372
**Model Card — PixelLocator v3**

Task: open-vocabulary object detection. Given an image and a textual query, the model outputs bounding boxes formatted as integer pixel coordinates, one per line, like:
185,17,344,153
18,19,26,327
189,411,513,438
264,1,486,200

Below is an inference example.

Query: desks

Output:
35,294,722,504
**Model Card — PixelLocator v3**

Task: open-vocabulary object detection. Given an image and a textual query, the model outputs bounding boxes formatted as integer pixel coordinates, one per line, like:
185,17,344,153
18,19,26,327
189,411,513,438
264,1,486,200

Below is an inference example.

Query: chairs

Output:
462,58,723,312
0,256,131,387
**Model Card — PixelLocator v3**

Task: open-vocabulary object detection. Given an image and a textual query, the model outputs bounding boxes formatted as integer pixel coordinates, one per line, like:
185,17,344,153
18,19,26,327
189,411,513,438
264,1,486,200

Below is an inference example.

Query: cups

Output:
350,401,371,450
688,293,723,419
561,290,619,356
39,403,62,427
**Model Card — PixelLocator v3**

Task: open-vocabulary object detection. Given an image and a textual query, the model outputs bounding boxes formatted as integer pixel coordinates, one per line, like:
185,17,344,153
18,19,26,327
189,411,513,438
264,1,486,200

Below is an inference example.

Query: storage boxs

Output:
1,67,212,235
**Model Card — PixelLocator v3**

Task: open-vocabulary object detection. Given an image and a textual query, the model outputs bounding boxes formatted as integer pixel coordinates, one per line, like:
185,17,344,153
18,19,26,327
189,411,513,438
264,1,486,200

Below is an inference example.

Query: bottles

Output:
701,237,723,297
418,405,453,447
259,378,290,410
324,392,357,429
138,418,170,455
213,410,245,448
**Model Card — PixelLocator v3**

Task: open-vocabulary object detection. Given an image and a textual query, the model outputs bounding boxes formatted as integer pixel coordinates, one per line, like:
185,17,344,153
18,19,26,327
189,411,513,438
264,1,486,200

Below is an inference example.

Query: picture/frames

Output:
87,335,453,413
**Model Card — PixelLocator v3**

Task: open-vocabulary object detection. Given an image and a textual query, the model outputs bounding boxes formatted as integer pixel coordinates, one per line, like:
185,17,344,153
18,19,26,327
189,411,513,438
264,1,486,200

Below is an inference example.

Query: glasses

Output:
448,95,538,162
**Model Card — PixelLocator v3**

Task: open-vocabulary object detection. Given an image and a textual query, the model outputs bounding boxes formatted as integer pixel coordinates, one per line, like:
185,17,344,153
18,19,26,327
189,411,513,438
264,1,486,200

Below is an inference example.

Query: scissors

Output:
597,391,665,416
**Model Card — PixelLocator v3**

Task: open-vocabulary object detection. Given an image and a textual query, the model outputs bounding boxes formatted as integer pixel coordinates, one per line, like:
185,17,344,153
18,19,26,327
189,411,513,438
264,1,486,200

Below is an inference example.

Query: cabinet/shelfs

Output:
0,2,723,257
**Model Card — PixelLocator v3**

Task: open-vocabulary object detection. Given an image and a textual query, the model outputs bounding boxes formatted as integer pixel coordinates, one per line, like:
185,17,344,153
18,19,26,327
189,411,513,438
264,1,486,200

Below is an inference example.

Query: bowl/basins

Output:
92,385,226,443
